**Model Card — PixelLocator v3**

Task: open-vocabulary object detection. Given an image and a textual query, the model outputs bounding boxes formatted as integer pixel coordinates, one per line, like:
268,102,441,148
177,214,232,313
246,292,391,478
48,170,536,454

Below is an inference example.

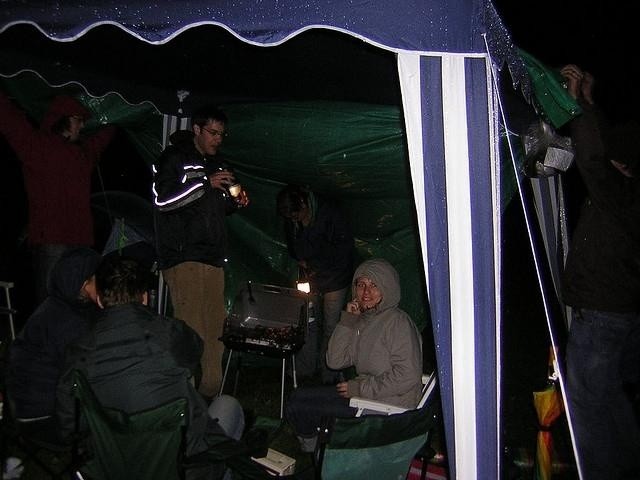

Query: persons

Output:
281,256,424,453
274,183,355,388
559,62,640,479
152,104,253,409
49,255,245,479
1,89,121,322
9,245,102,449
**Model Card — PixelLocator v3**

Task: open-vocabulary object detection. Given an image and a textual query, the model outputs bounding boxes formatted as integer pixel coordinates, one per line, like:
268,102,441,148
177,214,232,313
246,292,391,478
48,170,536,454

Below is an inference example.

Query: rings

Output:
571,69,575,75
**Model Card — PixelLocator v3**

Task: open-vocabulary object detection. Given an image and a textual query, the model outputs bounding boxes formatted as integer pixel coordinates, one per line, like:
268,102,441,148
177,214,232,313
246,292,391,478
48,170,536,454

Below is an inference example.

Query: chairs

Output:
312,368,443,480
2,277,20,342
217,281,315,420
73,384,267,479
0,392,95,480
157,271,168,317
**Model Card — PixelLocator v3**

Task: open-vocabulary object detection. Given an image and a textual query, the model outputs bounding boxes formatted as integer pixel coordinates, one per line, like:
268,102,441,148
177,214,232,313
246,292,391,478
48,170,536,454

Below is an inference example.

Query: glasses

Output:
201,126,229,138
